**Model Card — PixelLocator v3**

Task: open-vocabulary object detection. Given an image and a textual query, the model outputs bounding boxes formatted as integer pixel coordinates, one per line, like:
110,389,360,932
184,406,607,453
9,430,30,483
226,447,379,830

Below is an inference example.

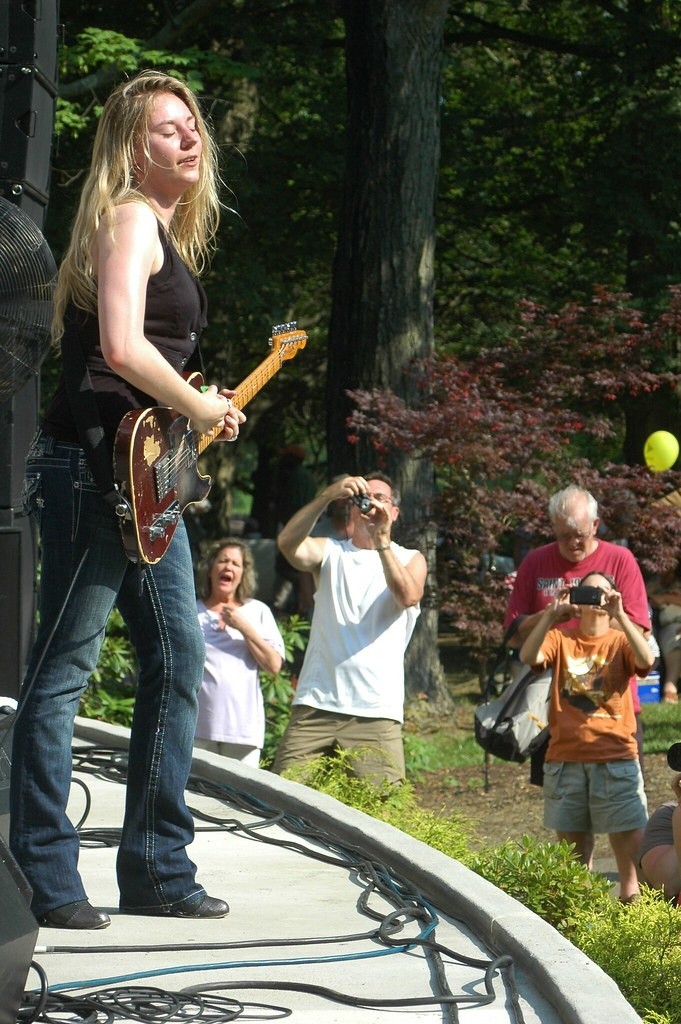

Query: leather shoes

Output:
119,886,232,920
39,893,113,932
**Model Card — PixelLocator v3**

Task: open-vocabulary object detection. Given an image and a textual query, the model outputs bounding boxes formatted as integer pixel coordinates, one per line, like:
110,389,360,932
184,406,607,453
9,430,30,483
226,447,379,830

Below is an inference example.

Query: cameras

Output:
352,493,375,515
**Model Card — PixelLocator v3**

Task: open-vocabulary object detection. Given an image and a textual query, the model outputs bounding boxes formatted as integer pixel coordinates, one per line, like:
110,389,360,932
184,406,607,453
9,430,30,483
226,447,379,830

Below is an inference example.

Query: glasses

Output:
362,492,396,505
553,530,592,541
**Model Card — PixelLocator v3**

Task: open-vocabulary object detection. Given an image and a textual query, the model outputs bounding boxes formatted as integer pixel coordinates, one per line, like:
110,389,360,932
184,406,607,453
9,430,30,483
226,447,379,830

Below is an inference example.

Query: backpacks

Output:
474,613,555,794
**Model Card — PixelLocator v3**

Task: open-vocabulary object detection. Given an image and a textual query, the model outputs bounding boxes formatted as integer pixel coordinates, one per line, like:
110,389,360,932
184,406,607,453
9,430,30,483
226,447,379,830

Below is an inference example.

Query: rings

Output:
228,435,237,441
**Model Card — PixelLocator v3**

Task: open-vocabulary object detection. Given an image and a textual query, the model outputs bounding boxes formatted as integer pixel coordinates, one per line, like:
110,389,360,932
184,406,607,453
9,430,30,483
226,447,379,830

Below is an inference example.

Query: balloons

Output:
644,430,679,473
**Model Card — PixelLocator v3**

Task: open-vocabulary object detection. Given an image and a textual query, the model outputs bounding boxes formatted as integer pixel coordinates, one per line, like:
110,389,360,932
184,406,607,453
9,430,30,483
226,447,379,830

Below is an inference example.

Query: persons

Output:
277,474,427,789
638,742,681,907
520,572,655,901
302,475,354,621
9,70,248,930
648,574,681,703
504,486,650,783
197,536,285,767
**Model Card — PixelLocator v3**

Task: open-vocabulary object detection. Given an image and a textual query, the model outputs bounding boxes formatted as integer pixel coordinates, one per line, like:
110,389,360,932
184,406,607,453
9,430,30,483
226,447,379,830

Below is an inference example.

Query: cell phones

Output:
570,586,602,606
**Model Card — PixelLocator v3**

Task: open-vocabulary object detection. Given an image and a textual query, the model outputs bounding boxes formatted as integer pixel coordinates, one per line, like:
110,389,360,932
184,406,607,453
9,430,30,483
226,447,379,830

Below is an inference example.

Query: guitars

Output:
110,318,308,570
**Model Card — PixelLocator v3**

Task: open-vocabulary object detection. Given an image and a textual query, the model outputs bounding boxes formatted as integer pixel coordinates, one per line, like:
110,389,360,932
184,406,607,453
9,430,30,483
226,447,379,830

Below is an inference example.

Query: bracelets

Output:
376,546,390,551
